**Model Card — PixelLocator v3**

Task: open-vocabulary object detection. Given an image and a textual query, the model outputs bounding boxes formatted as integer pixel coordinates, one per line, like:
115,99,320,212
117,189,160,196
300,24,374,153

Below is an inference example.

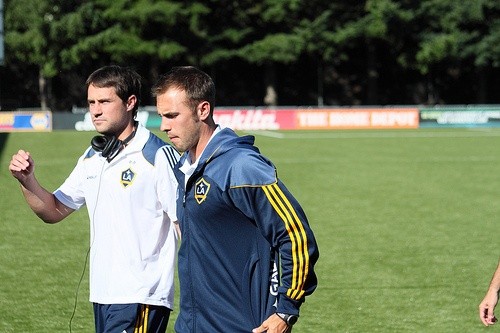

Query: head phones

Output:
91,121,139,160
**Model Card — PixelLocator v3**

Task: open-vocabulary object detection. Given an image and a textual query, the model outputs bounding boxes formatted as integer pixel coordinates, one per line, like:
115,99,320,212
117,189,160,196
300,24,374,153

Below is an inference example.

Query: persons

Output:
9,65,181,333
151,66,320,333
478,259,500,327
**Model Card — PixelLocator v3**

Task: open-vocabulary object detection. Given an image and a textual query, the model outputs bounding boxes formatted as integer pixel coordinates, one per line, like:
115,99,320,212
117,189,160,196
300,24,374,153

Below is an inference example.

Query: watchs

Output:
276,312,298,327
